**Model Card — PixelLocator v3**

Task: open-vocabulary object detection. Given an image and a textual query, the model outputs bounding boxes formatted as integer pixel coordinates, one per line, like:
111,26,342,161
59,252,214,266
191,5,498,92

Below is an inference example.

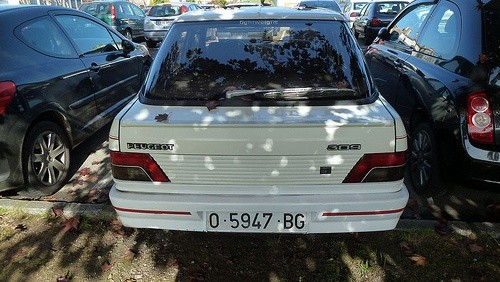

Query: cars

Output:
365,0,500,196
0,6,153,197
144,2,222,48
78,0,156,43
293,0,353,29
343,2,410,45
110,6,409,236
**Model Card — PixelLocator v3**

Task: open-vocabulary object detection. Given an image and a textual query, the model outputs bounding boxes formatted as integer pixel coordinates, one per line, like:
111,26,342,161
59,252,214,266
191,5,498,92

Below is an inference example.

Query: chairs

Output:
389,6,400,14
442,14,457,54
